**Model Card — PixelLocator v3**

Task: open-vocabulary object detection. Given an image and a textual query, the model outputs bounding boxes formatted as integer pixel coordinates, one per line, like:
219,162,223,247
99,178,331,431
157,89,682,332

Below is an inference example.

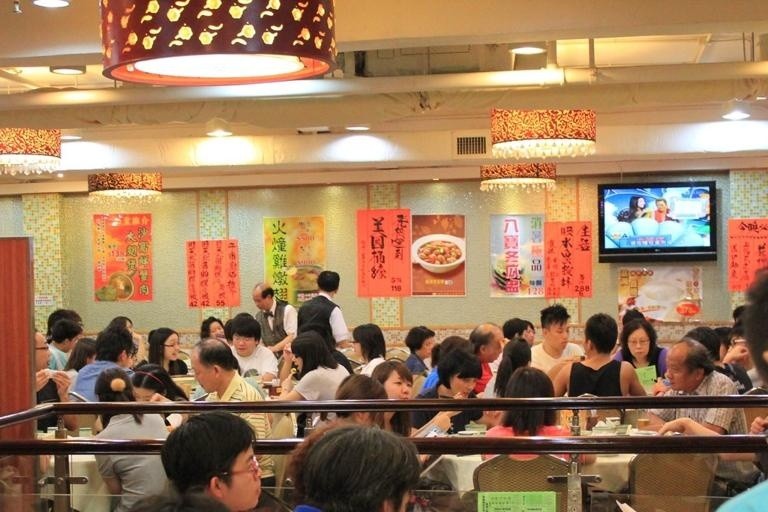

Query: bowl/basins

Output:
412,233,466,274
641,280,688,305
590,423,632,436
108,272,134,301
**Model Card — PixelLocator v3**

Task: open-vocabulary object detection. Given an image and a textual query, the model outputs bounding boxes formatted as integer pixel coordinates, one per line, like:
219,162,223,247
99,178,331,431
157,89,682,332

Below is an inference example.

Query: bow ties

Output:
262,311,273,318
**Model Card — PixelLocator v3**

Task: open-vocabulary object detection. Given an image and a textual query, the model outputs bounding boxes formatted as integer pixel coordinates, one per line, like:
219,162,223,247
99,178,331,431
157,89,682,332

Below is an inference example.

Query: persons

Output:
655,198,671,222
627,196,647,222
36,269,768,510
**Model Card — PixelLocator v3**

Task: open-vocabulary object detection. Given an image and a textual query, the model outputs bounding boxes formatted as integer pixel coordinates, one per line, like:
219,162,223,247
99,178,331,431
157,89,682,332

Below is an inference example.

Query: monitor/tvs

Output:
598,180,717,263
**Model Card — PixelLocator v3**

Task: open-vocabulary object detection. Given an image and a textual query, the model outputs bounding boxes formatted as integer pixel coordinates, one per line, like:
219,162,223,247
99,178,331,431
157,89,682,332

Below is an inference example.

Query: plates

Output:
263,382,272,386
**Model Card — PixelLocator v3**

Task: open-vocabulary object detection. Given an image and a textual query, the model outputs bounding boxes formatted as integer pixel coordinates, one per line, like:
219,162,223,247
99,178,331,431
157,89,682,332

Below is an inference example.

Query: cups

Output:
465,424,487,431
47,426,56,439
78,427,93,439
586,416,599,430
270,386,281,396
570,425,580,435
458,430,472,434
637,408,651,431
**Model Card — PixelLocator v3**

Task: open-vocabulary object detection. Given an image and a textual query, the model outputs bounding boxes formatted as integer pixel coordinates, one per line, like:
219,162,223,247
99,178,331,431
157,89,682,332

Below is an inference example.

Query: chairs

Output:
35,329,768,511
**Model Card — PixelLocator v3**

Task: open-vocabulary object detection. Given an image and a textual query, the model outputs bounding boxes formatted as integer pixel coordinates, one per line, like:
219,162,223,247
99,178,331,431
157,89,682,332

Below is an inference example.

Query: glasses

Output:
220,457,259,477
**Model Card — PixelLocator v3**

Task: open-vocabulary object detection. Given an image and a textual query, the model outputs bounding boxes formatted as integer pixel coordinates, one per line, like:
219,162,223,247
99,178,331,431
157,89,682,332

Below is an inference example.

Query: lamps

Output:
489,107,596,162
479,160,557,193
87,171,163,203
97,0,339,86
0,127,63,177
